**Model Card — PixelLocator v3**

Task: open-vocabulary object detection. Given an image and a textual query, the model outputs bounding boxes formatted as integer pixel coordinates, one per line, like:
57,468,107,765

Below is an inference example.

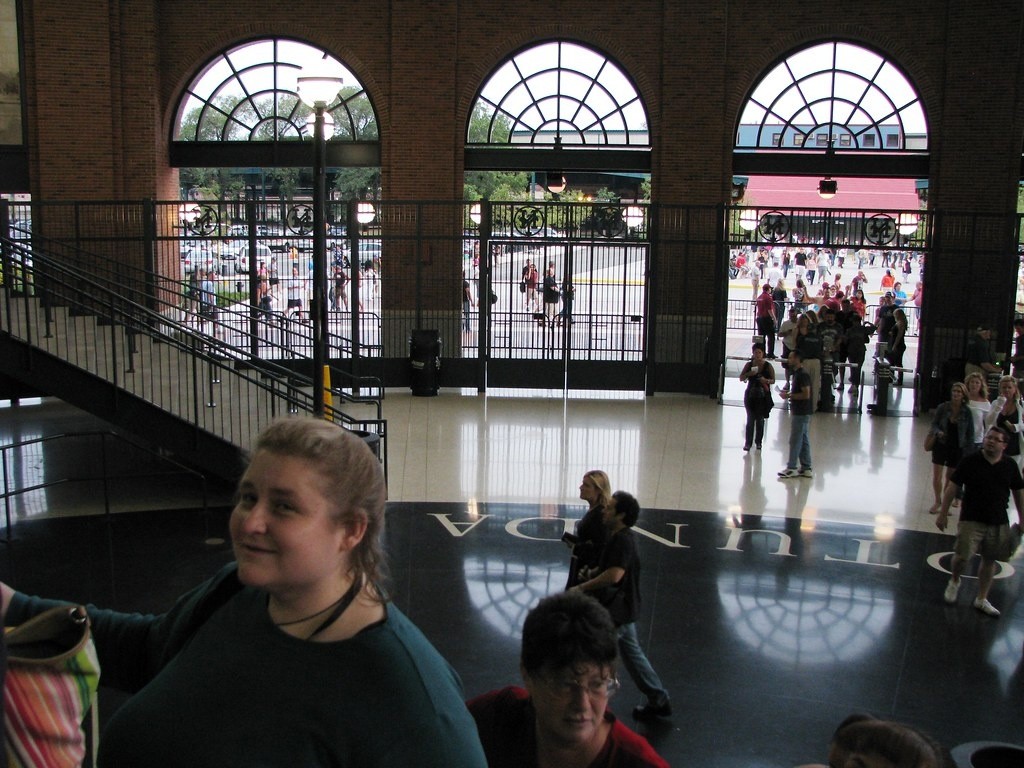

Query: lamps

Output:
817,177,838,195
547,172,564,186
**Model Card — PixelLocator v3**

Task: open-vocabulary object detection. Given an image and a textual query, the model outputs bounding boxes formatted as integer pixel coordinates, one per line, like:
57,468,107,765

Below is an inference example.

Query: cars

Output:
179,224,272,276
10,218,32,267
342,243,382,268
257,225,346,252
463,227,558,253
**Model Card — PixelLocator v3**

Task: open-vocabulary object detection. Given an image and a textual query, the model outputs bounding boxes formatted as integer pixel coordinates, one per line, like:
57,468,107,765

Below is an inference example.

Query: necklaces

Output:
276,587,345,626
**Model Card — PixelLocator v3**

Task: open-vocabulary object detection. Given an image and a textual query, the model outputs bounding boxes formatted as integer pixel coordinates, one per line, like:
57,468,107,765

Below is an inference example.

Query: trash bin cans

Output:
409,328,442,397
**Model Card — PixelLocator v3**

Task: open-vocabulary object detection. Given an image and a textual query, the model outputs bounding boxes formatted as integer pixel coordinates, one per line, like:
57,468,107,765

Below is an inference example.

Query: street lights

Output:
296,59,343,418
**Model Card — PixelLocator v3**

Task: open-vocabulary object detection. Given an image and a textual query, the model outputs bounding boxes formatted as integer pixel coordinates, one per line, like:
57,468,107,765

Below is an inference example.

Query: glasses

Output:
984,435,1004,443
541,670,620,701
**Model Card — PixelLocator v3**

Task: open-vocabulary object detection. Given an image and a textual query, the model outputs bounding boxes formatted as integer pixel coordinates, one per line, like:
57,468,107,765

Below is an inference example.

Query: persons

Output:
565,470,673,719
183,240,576,334
730,233,1024,617
830,712,957,768
0,422,488,768
466,591,670,768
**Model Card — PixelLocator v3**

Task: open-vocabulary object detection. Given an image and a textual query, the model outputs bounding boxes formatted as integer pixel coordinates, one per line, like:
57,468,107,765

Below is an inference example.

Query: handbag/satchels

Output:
520,281,525,293
925,433,937,452
0,604,101,768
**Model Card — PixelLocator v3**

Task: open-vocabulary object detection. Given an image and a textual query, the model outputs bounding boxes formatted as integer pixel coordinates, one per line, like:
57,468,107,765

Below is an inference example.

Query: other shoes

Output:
894,380,904,386
767,353,778,359
952,499,960,507
837,382,844,390
756,443,762,449
930,499,942,513
743,443,752,451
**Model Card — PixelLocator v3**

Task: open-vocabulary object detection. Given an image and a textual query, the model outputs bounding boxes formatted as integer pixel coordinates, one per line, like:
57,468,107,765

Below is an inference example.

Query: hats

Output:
764,284,774,289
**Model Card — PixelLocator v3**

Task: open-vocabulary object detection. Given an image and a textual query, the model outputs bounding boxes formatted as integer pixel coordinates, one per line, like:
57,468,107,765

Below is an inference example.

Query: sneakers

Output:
777,467,799,477
632,700,672,720
799,469,813,476
973,596,1000,615
944,577,962,602
